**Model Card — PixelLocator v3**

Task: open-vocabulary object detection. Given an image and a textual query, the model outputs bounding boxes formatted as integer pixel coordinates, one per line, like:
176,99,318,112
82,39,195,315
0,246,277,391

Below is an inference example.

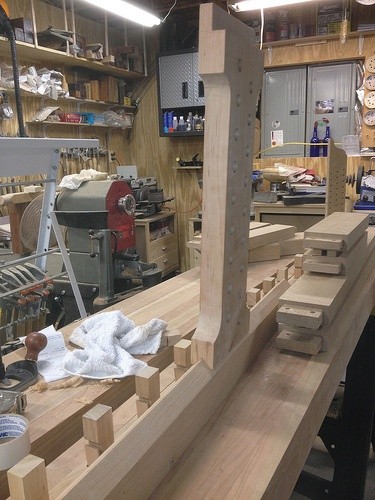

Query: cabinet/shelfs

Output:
156,47,205,137
0,0,148,127
135,211,179,278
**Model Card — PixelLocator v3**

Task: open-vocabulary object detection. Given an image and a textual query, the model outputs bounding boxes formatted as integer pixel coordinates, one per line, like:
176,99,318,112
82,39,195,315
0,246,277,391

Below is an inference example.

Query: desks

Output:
252,199,354,225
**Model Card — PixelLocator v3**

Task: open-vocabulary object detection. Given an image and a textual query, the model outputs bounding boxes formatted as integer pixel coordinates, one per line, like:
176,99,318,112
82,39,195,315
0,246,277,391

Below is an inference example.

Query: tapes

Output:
0,414,30,470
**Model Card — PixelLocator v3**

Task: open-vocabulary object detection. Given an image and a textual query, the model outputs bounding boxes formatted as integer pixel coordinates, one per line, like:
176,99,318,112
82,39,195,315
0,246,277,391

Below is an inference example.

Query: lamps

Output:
231,0,308,12
87,0,162,27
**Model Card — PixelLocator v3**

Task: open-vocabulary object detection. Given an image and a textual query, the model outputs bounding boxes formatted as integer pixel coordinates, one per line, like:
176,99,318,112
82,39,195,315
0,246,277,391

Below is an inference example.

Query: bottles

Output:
310,128,319,157
163,111,205,133
323,127,331,157
